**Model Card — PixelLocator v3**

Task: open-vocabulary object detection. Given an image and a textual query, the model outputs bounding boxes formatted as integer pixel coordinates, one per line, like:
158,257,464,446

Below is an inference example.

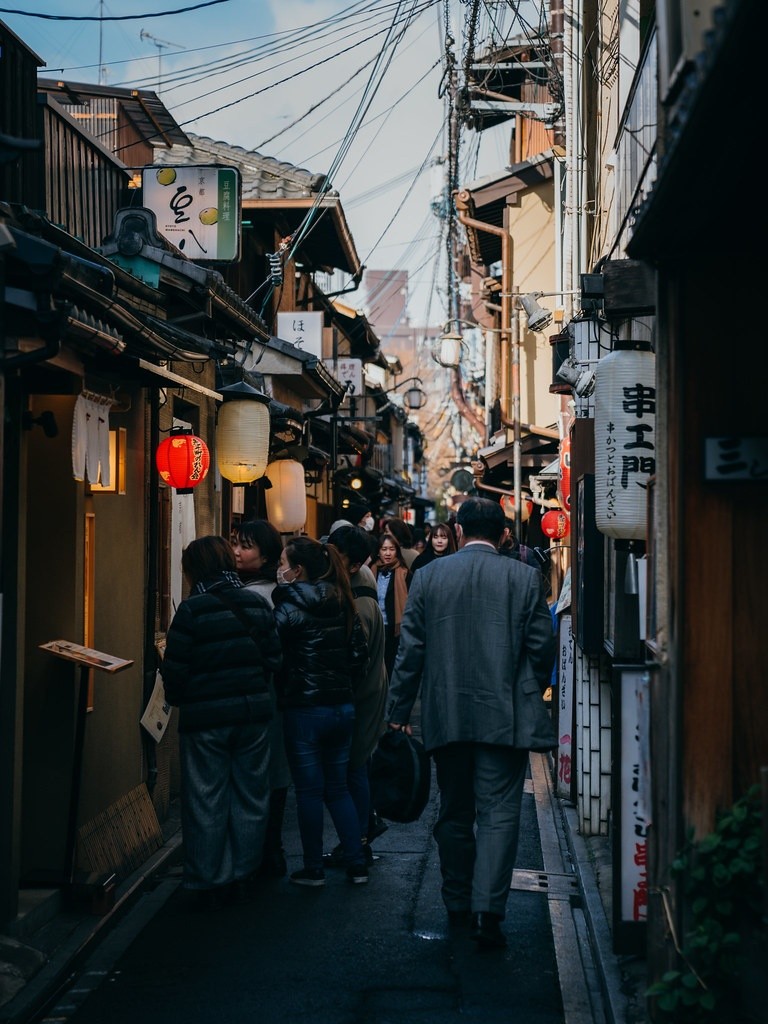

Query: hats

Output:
346,503,371,526
330,520,353,535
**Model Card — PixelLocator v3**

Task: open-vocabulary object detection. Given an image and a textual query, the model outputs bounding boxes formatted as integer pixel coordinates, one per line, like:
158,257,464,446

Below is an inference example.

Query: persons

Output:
384,498,553,945
162,506,420,896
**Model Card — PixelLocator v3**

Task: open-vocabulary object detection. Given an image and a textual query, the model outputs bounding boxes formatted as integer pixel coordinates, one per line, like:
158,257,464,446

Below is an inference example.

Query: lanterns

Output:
500,490,533,523
156,429,210,495
595,341,655,552
560,438,570,511
218,400,270,487
542,507,569,542
265,457,306,536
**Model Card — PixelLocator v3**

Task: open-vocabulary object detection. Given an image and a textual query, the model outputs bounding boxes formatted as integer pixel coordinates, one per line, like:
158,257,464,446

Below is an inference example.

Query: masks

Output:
359,517,375,531
277,568,296,585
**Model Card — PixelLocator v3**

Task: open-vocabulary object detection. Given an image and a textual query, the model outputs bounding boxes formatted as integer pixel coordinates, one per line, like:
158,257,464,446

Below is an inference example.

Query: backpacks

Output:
368,729,432,824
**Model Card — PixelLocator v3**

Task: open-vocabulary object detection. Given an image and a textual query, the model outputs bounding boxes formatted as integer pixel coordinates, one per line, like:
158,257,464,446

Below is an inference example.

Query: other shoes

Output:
228,884,250,905
266,848,288,875
448,913,473,940
470,913,506,946
323,842,374,865
360,814,388,843
290,866,325,886
199,887,222,913
348,865,369,885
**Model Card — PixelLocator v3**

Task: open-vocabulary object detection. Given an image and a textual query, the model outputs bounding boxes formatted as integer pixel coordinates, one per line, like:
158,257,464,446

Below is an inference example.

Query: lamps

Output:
430,318,507,368
84,427,128,499
533,544,576,565
343,377,425,410
519,293,554,332
24,408,59,437
557,358,594,399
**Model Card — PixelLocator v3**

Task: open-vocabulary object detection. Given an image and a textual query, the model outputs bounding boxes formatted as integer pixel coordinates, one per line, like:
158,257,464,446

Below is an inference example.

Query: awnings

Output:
0,202,437,510
470,425,559,507
